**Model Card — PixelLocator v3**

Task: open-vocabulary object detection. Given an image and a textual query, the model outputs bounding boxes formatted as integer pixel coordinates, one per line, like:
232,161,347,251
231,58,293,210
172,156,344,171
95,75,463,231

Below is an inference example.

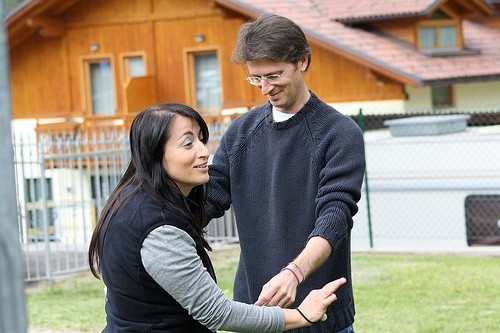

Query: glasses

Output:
245,62,290,86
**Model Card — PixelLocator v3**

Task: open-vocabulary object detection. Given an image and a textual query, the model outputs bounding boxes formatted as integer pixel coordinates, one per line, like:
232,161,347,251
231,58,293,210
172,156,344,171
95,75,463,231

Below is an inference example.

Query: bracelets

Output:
296,308,321,324
289,261,306,284
280,267,300,286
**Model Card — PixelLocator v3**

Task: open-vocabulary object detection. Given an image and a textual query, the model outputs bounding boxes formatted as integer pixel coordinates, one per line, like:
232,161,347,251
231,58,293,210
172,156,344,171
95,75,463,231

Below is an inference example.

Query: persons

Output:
201,13,366,333
83,102,347,333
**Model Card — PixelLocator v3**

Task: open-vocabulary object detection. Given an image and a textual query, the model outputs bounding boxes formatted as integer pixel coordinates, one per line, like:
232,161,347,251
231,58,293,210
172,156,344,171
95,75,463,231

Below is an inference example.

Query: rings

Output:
278,304,282,308
